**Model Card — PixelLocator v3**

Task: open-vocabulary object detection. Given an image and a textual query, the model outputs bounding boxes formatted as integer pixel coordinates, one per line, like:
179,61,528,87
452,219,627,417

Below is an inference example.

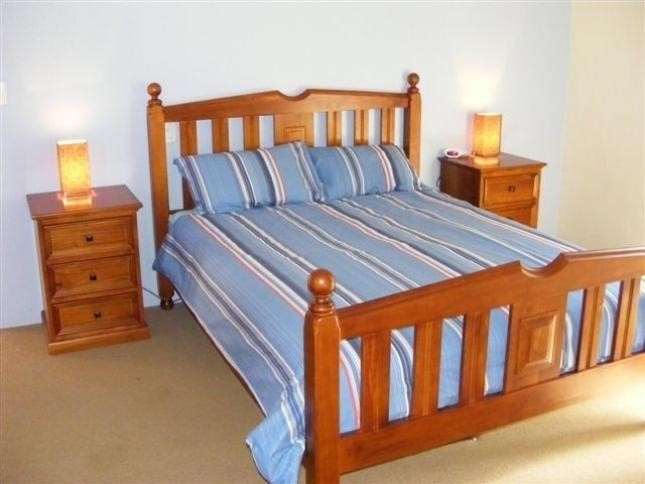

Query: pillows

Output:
173,140,419,214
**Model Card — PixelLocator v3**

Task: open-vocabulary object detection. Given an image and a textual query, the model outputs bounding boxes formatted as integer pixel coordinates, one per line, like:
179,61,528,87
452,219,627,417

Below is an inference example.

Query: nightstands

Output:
24,185,149,356
438,151,547,231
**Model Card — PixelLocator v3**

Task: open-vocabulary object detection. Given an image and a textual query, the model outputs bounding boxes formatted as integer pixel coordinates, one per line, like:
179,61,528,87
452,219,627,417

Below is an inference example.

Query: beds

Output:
146,72,645,484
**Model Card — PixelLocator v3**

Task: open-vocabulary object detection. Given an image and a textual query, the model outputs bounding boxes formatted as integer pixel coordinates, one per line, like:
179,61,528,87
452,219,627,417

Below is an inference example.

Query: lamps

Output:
472,109,502,166
53,139,97,207
